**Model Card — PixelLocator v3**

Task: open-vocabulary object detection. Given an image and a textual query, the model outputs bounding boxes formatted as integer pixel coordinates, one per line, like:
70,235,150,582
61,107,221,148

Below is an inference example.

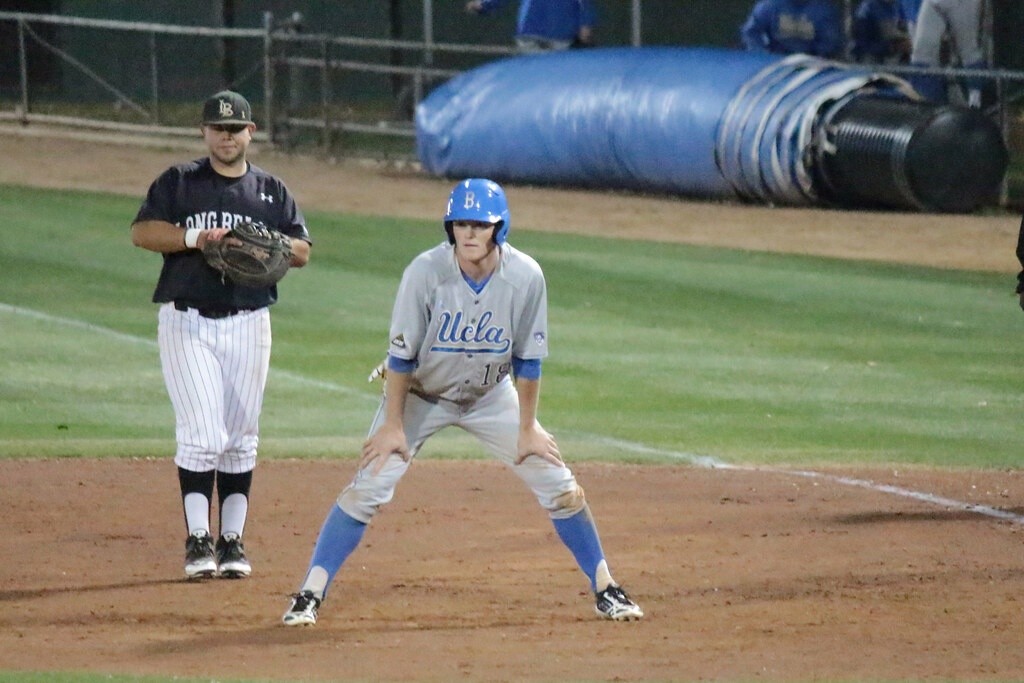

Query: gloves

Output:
203,220,294,289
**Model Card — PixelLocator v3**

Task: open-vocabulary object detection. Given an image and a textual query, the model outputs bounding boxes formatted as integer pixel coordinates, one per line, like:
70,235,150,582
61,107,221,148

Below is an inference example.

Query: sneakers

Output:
184,528,217,580
594,582,644,621
215,531,252,578
282,589,321,627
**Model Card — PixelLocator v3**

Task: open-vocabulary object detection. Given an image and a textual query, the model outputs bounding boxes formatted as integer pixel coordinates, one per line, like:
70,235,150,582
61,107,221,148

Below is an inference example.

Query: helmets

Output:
443,178,511,248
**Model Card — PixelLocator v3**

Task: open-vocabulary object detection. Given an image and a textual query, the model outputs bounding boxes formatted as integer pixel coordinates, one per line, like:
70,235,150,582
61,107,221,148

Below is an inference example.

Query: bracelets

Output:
184,229,201,247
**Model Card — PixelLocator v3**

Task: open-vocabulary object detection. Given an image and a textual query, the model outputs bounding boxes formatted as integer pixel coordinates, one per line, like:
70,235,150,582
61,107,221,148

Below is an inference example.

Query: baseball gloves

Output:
202,219,294,284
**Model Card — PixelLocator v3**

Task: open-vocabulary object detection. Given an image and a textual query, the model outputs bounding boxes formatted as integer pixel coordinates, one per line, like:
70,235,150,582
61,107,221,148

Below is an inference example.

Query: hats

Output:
202,89,255,126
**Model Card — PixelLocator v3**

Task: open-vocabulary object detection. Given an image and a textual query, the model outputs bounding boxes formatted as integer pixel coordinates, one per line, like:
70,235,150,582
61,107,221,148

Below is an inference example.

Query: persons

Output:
130,90,311,580
741,0,839,60
852,0,983,109
464,0,593,51
284,177,644,625
